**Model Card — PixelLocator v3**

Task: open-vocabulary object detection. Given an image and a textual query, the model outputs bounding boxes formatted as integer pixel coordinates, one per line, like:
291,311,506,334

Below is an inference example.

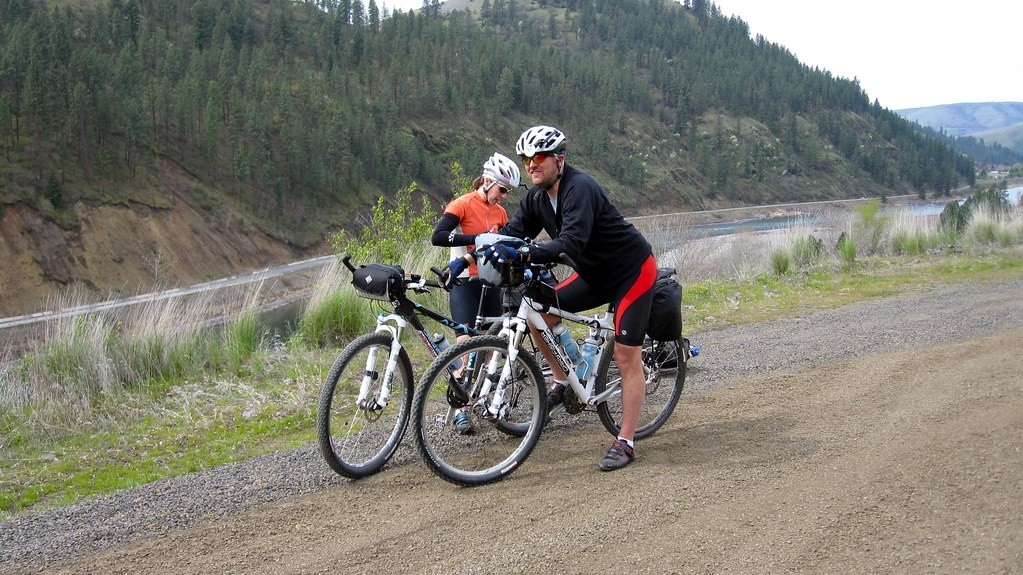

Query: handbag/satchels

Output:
645,267,683,340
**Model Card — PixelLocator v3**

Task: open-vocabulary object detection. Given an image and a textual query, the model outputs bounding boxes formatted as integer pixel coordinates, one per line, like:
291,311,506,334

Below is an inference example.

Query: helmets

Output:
482,152,520,189
516,126,567,157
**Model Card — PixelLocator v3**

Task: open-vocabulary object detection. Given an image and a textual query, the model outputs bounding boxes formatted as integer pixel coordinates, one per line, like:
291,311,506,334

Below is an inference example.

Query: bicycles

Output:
316,242,699,488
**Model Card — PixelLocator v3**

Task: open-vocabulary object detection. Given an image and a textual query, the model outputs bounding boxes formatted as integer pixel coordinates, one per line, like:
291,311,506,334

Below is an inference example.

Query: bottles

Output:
553,323,584,367
432,333,464,369
575,336,600,380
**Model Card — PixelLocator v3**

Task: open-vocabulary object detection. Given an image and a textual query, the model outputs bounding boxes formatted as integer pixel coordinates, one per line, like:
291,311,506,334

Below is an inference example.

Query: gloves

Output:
476,243,519,272
437,257,468,292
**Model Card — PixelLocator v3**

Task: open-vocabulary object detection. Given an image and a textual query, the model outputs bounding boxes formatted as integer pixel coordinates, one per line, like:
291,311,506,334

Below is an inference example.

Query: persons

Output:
431,153,519,434
437,125,658,471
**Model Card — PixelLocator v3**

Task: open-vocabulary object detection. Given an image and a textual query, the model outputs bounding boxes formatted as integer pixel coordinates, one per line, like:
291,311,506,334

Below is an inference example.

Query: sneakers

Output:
597,436,634,470
453,410,475,434
546,380,566,414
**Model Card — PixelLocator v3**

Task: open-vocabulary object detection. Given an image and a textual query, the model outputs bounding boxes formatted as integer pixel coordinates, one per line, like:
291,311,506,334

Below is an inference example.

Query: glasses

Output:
494,182,512,194
522,153,556,164
